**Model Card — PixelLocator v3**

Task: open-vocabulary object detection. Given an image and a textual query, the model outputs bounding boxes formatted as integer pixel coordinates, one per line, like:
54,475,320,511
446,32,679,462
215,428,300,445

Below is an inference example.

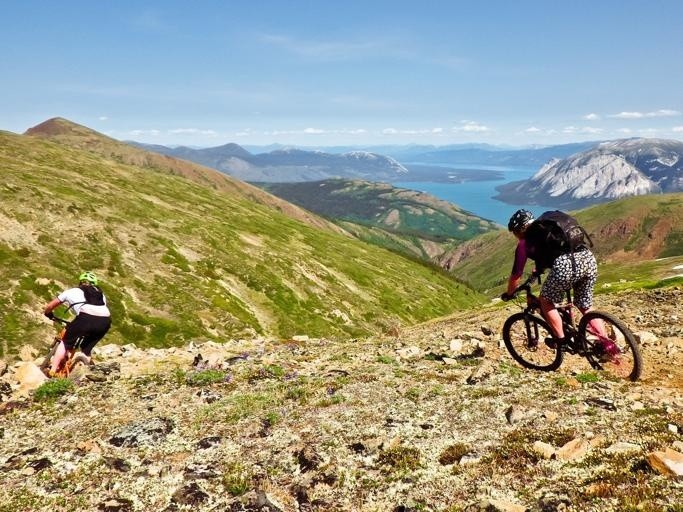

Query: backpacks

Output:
79,284,106,305
525,209,584,254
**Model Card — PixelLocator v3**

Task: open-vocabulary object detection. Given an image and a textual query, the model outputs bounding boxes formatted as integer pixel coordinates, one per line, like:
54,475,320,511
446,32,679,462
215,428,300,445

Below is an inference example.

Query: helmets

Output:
80,270,98,286
507,208,534,232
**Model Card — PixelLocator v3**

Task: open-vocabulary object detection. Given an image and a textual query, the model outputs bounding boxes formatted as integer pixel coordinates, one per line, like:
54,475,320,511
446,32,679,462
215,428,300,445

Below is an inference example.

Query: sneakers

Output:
544,337,568,351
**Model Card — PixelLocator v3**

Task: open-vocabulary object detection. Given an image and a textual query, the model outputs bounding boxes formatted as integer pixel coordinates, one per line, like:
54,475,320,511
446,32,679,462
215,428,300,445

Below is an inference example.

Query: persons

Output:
502,208,615,349
38,271,114,377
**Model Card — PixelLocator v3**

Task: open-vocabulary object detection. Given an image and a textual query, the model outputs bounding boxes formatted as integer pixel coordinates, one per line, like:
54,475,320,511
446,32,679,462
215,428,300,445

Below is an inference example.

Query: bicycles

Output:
41,313,92,380
499,269,646,384
